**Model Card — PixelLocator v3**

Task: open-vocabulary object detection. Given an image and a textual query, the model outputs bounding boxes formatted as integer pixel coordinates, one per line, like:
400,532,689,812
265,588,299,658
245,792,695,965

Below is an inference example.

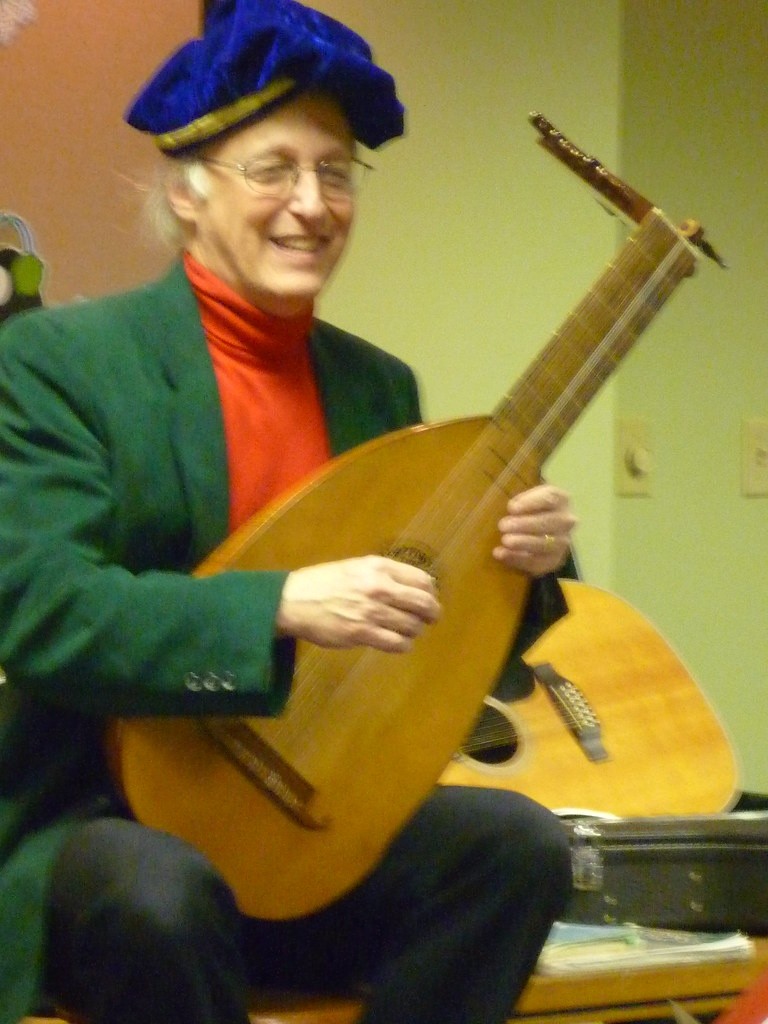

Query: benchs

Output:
21,951,755,1023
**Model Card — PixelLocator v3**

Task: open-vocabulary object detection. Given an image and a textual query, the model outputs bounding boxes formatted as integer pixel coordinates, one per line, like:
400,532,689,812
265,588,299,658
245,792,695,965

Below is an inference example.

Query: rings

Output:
544,534,555,554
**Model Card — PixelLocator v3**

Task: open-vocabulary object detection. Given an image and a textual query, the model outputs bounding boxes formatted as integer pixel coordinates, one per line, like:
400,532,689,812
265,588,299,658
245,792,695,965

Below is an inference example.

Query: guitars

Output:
433,575,744,818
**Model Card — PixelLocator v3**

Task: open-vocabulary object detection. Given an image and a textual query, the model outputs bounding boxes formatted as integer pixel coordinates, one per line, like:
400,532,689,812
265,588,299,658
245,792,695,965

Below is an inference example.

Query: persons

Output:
0,0,579,1024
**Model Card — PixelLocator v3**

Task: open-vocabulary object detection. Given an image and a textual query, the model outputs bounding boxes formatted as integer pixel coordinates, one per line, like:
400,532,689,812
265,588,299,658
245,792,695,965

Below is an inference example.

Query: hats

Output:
122,0,404,157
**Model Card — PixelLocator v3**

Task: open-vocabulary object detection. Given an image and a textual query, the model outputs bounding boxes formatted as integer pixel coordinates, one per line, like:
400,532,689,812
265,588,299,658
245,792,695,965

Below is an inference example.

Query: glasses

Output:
188,157,375,196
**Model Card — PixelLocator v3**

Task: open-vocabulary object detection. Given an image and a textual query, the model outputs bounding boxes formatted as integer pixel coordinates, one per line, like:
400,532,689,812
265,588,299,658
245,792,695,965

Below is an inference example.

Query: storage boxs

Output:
563,818,768,935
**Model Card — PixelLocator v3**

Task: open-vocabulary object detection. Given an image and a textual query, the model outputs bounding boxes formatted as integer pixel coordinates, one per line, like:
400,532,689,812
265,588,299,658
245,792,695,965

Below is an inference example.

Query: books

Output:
535,923,754,968
